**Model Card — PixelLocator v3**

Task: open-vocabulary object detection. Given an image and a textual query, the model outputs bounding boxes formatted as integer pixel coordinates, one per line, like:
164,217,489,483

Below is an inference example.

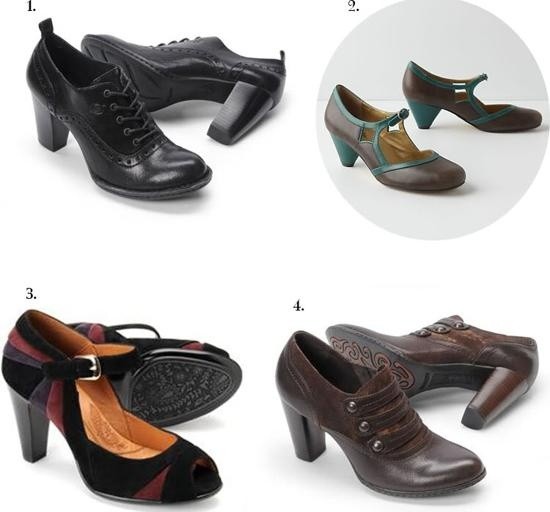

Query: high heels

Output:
25,18,213,199
324,59,543,194
66,322,243,425
80,34,288,145
0,309,222,505
275,330,487,498
326,314,539,430
